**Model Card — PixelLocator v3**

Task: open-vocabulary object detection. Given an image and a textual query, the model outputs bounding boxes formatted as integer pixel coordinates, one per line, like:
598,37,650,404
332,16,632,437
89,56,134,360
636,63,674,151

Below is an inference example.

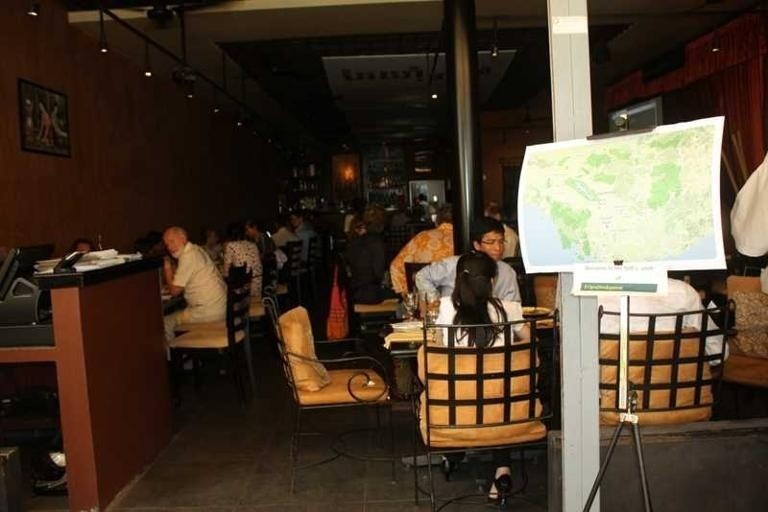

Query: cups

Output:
425,292,439,322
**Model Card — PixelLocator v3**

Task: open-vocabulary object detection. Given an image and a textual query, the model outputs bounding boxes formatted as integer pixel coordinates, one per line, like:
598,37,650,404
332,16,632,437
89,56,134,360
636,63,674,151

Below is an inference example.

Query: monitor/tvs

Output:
0,238,58,302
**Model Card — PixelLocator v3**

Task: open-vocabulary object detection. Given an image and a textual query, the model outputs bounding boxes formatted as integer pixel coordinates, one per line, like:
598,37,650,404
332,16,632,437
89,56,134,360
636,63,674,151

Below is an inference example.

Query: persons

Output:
484,201,519,258
389,203,455,311
162,225,229,367
219,221,262,314
340,194,388,290
388,189,439,245
415,217,539,341
599,271,730,366
245,203,318,294
70,239,96,252
416,250,540,511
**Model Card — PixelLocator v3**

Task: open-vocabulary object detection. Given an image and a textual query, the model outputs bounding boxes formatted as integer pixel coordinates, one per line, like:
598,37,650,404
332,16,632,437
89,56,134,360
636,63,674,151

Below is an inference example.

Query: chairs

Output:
169,233,328,402
412,316,545,511
597,297,736,422
262,297,401,495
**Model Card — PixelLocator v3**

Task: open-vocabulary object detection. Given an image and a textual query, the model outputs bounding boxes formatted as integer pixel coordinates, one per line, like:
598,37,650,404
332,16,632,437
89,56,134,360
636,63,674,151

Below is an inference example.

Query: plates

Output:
392,322,422,330
522,307,551,318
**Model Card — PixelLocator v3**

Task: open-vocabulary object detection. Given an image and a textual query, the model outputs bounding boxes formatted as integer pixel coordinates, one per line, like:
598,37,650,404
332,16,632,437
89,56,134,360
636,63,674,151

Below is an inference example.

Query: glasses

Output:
481,239,508,245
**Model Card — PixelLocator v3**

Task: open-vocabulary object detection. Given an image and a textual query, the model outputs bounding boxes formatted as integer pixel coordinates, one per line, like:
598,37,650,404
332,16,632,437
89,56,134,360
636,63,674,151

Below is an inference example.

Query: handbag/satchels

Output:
326,264,348,342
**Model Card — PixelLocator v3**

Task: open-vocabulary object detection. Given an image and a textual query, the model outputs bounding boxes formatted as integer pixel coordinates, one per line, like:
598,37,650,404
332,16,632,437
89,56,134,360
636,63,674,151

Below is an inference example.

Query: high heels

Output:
442,456,457,482
487,474,513,504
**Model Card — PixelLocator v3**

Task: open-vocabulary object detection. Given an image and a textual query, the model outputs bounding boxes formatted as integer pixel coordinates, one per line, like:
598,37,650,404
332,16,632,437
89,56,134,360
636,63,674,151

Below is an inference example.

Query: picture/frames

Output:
16,78,72,158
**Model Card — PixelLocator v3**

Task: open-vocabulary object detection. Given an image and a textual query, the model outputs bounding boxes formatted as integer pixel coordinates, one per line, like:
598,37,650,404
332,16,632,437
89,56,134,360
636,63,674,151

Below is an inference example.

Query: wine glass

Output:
404,293,418,323
